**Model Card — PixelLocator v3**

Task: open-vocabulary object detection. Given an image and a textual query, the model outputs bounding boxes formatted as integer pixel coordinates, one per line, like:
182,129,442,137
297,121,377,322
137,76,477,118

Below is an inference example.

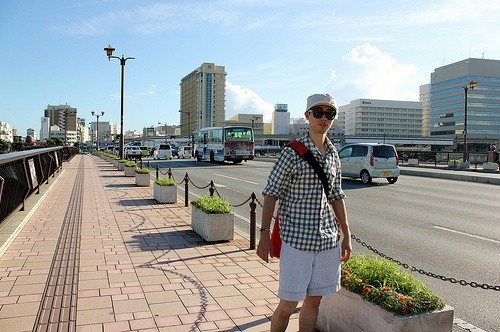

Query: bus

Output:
194,125,254,165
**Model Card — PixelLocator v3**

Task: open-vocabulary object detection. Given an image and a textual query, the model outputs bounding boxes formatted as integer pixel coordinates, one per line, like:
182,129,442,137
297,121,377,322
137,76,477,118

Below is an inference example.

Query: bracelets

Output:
259,228,270,233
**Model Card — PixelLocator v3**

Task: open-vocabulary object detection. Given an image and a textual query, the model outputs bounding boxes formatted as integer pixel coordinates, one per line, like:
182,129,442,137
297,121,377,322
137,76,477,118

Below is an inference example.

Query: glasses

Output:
308,109,336,120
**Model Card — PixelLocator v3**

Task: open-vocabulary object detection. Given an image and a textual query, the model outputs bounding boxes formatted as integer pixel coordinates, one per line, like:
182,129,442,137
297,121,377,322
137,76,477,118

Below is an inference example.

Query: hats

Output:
305,93,337,112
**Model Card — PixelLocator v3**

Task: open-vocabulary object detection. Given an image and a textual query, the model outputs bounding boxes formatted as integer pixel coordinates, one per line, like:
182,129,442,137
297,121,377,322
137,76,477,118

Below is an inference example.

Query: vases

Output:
318,283,455,332
408,159,418,164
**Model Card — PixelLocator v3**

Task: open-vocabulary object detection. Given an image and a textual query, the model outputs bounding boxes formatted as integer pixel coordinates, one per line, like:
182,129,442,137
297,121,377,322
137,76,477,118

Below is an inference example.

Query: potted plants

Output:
154,178,178,203
448,160,459,166
97,151,151,187
190,195,235,242
483,162,499,170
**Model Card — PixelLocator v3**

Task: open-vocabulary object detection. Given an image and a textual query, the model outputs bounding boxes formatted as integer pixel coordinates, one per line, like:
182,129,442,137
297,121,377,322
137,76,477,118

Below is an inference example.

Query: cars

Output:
99,140,153,161
167,143,179,156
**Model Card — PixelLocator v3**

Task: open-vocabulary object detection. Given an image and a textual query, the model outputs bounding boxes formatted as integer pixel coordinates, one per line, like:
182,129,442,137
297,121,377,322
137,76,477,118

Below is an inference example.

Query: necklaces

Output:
317,142,327,149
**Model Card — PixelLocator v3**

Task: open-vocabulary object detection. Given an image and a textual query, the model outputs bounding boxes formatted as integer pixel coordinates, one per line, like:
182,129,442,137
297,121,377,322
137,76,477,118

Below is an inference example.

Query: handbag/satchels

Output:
270,211,282,258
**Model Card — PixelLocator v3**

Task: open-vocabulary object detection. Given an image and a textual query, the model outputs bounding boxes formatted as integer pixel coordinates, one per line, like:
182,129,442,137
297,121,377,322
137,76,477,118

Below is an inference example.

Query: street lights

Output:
91,111,105,151
463,79,478,162
158,122,167,139
252,116,261,128
104,44,135,160
179,110,190,147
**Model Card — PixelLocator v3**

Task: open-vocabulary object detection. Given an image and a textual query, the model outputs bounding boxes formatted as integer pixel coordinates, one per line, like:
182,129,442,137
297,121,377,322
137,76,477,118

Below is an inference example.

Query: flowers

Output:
340,251,445,313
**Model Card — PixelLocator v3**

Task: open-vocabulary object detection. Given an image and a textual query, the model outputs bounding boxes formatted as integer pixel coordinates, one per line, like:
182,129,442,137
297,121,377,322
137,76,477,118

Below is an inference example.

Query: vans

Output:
337,142,401,185
152,143,174,159
177,146,192,159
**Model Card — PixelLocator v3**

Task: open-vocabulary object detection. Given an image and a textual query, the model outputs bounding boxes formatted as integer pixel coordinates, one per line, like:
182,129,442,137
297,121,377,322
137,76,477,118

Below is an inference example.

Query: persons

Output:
257,93,353,332
